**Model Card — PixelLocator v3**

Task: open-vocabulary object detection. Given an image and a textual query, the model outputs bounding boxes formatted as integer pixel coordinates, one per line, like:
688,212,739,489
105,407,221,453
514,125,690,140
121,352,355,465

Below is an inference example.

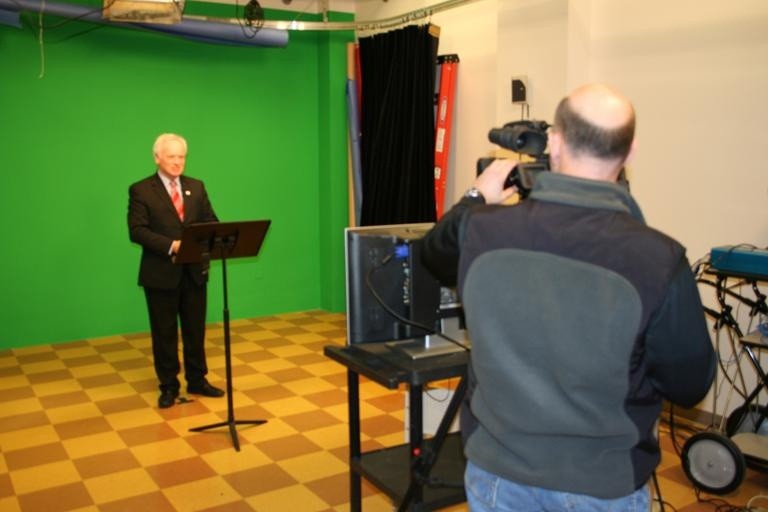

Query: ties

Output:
170,179,184,223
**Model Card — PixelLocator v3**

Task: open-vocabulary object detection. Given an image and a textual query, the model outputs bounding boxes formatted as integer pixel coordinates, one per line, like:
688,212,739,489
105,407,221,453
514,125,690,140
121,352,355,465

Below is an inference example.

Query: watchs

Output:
463,188,485,207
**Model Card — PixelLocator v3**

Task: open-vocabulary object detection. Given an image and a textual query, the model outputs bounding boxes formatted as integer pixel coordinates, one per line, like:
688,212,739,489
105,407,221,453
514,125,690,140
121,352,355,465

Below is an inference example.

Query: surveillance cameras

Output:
244,0,264,34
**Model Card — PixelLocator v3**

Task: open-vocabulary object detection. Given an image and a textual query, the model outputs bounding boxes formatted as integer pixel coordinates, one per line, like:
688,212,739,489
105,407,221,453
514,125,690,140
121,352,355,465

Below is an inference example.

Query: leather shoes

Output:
159,387,180,408
187,380,225,397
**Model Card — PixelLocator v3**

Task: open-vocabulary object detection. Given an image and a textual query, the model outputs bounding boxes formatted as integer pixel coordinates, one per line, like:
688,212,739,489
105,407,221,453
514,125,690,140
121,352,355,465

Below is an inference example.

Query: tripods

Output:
187,260,269,452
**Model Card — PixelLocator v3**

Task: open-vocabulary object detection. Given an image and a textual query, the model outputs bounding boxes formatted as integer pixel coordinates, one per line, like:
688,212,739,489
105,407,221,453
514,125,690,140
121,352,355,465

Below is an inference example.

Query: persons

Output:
126,133,223,409
420,85,716,512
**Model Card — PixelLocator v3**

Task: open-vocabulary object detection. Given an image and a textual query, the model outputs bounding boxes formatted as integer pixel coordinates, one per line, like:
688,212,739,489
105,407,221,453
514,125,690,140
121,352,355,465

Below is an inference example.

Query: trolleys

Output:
680,274,767,496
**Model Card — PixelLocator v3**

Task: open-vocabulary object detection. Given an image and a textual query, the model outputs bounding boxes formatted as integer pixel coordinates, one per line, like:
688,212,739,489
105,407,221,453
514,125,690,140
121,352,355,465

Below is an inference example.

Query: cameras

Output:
476,118,555,202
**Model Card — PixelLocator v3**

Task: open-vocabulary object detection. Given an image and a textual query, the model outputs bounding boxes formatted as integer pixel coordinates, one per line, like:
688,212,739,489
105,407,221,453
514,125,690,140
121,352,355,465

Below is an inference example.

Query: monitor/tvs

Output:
344,221,463,360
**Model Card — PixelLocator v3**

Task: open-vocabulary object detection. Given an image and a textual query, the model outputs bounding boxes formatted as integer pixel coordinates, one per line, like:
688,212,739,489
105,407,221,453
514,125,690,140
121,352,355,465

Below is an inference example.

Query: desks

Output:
323,346,468,512
